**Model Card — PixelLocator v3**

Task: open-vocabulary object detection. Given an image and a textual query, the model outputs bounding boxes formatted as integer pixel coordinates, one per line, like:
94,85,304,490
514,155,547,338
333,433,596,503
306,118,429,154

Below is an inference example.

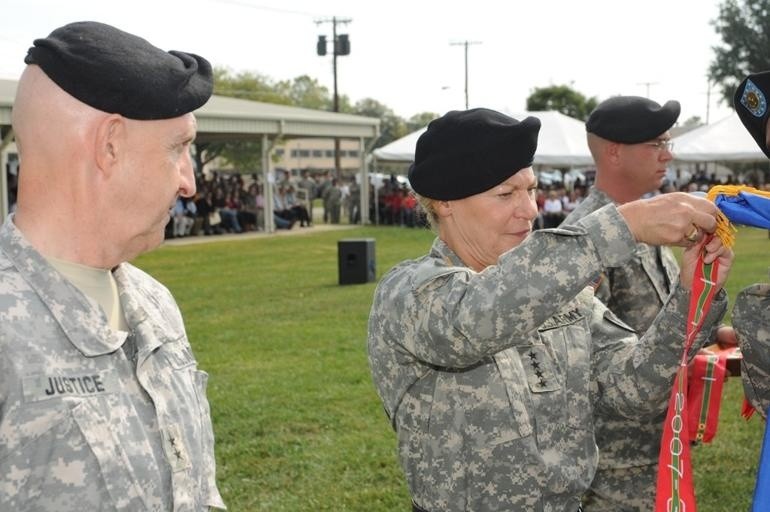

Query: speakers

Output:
338,240,375,284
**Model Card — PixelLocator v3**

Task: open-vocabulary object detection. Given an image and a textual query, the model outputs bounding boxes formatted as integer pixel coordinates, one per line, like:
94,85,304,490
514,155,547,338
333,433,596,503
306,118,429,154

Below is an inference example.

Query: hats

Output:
732,71,770,157
584,95,682,145
23,21,214,120
407,106,543,201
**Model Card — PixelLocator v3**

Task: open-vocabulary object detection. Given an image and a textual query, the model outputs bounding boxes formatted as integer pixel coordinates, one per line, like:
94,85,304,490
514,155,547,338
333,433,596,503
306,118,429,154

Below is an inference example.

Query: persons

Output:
3,18,227,512
656,170,760,194
7,162,20,215
558,96,738,510
534,180,588,228
367,106,729,510
164,158,430,237
